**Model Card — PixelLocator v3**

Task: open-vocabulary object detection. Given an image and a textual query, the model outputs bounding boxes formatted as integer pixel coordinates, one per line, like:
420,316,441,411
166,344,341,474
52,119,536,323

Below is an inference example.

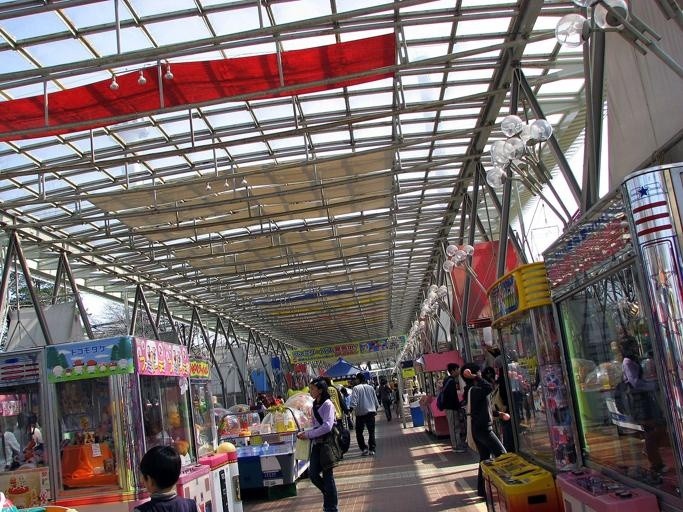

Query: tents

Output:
315,358,372,382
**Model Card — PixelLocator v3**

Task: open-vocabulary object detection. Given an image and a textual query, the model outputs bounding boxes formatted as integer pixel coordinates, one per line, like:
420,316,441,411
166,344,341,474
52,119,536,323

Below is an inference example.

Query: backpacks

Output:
437,378,454,412
391,389,396,400
614,366,635,416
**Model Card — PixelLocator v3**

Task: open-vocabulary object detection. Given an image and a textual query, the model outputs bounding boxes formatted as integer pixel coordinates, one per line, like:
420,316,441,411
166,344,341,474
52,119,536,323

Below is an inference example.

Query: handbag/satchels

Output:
614,361,644,416
465,385,482,454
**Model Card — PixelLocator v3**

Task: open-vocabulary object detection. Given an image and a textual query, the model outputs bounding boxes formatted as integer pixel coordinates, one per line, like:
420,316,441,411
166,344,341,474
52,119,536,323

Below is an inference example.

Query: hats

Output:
494,355,504,368
393,383,398,389
483,366,496,378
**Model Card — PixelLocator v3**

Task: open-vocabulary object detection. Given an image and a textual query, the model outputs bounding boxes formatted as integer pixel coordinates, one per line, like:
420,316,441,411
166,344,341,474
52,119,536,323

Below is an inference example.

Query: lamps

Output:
443,244,489,297
555,1,683,80
484,114,572,230
425,285,458,327
420,299,449,347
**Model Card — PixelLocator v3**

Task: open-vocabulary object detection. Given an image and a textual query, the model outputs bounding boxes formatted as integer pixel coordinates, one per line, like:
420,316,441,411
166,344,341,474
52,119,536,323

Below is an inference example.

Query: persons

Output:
460,362,507,502
511,379,524,419
492,349,508,405
378,379,392,422
131,446,201,512
482,367,495,383
413,376,419,395
442,363,467,453
341,387,353,430
321,377,344,461
491,367,514,453
373,381,380,393
391,383,404,417
648,352,655,372
348,372,380,456
614,335,670,473
299,378,338,512
257,393,270,416
278,395,284,404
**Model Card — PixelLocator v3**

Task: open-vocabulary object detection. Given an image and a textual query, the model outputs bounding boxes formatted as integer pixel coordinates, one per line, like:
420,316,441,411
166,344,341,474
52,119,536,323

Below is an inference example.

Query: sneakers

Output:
361,450,369,456
369,450,376,455
